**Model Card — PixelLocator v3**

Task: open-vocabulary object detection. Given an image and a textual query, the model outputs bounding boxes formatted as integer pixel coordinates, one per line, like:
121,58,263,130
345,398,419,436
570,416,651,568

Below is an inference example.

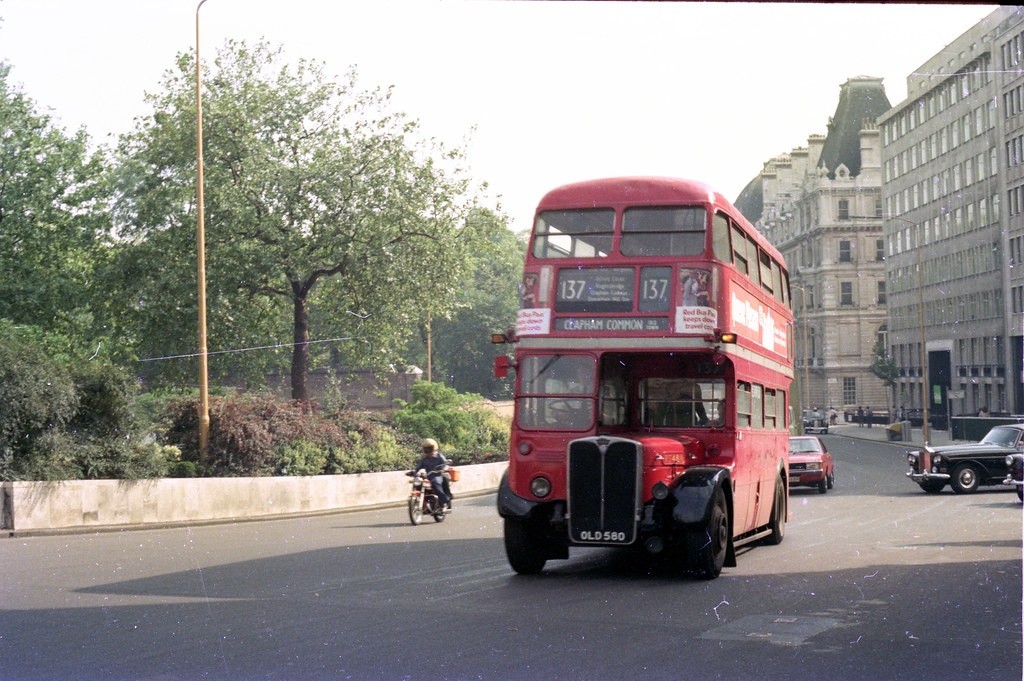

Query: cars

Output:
776,436,834,491
799,408,831,434
906,426,1024,495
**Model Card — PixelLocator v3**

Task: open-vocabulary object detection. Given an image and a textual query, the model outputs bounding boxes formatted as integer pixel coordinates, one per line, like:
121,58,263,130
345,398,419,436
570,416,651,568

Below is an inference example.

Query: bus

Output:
497,174,798,580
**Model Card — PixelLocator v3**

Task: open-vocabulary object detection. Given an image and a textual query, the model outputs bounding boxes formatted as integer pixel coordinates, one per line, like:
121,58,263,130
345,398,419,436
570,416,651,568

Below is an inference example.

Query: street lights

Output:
789,280,813,429
883,214,931,448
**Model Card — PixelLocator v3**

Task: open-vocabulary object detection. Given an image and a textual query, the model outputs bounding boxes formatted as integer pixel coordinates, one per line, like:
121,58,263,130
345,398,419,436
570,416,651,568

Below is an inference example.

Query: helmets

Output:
422,438,438,457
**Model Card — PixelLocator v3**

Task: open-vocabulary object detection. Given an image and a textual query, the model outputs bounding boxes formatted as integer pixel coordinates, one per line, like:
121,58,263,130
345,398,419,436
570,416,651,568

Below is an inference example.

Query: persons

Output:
978,407,990,417
707,400,725,428
857,406,865,428
562,363,614,428
866,406,873,429
891,406,898,422
827,407,838,426
415,438,450,514
897,405,905,422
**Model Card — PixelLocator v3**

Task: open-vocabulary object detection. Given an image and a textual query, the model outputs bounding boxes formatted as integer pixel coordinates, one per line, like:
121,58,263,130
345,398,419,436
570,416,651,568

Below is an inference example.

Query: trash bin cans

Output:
900,420,911,442
847,414,853,422
885,422,903,441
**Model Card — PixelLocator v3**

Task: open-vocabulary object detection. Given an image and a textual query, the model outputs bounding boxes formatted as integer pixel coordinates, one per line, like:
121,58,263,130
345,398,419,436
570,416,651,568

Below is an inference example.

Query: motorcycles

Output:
407,469,458,522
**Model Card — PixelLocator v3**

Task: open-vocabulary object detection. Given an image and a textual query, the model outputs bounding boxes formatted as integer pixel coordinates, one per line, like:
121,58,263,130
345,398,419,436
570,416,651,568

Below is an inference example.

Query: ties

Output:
581,385,588,409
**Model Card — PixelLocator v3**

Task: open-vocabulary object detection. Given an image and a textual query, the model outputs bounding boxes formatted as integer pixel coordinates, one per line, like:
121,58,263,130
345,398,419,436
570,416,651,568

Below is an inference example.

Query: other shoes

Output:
442,503,448,512
